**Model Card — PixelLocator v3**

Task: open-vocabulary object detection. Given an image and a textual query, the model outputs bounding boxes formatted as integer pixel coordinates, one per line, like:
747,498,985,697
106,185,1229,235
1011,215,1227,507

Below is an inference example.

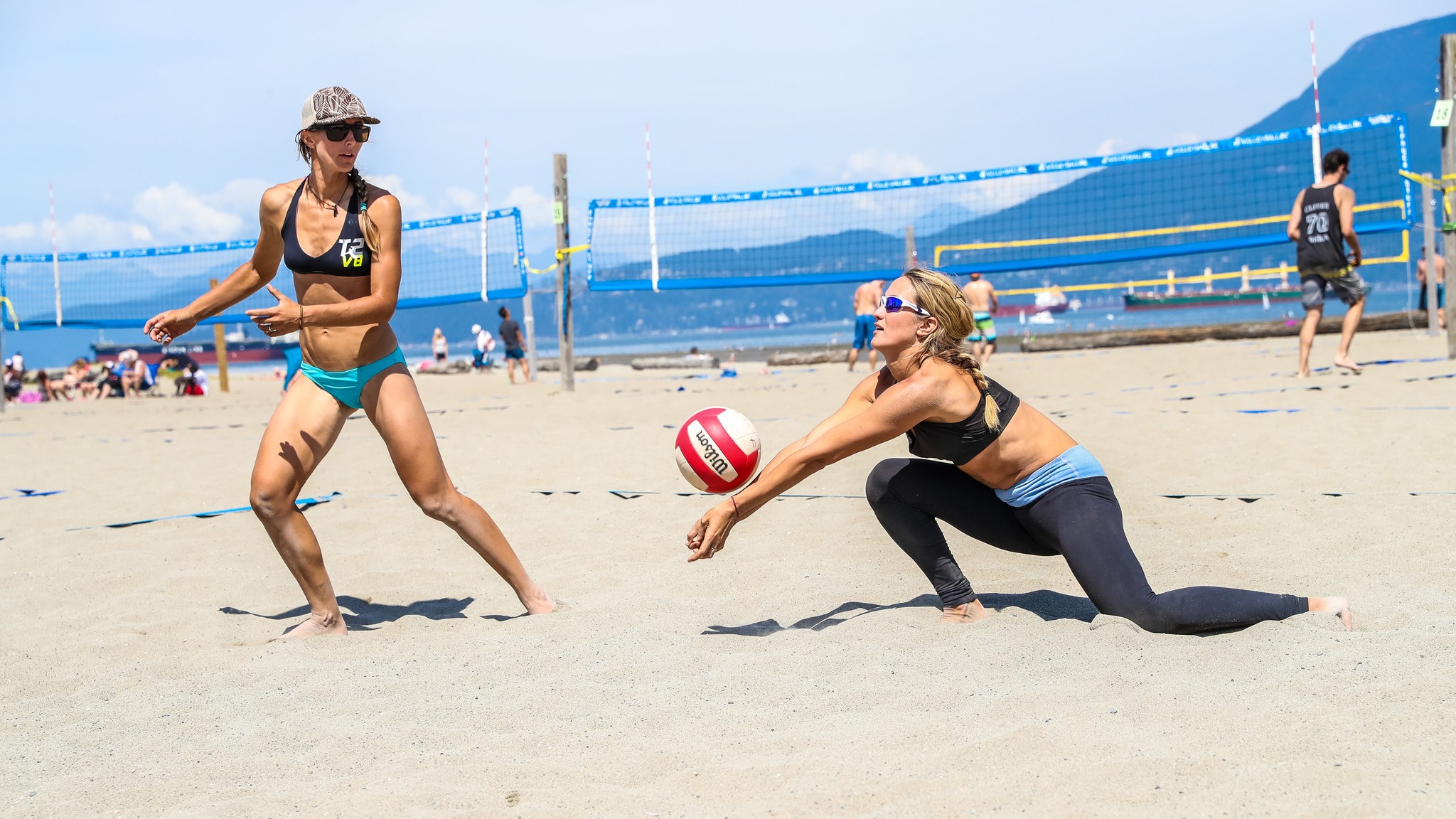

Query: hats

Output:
471,324,481,334
301,86,381,131
5,358,13,367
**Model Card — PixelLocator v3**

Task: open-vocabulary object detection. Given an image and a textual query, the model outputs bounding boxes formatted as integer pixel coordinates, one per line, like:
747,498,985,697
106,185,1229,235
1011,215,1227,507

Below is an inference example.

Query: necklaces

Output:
308,173,350,217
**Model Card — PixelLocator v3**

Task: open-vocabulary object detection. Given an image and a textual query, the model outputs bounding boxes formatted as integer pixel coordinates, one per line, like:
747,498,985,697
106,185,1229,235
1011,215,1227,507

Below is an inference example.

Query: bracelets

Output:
731,495,739,518
299,304,303,331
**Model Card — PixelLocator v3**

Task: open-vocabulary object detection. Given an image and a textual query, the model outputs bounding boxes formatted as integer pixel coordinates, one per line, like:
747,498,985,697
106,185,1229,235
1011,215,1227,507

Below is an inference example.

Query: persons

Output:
431,328,449,363
686,267,1354,634
497,306,531,384
142,85,550,636
1416,242,1447,331
472,324,496,373
849,279,884,372
962,272,998,365
1287,148,1368,377
1,348,208,400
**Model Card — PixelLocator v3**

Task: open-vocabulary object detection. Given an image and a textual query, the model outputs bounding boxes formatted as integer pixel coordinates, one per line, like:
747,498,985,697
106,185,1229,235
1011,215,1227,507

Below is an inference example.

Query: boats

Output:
1123,261,1377,309
91,323,299,366
990,301,1070,318
723,320,792,330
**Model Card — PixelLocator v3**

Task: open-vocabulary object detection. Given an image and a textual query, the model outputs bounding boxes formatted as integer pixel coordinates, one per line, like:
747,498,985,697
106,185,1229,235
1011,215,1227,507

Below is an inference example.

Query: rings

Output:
269,325,273,333
709,546,717,551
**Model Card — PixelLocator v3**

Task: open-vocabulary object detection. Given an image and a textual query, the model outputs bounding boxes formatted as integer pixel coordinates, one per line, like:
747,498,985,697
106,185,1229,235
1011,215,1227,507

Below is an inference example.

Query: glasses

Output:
878,294,932,318
306,124,371,143
1343,168,1350,174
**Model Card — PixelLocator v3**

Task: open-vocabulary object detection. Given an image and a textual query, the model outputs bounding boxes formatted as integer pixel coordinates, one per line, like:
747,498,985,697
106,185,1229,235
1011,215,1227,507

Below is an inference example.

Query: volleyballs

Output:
673,405,762,495
